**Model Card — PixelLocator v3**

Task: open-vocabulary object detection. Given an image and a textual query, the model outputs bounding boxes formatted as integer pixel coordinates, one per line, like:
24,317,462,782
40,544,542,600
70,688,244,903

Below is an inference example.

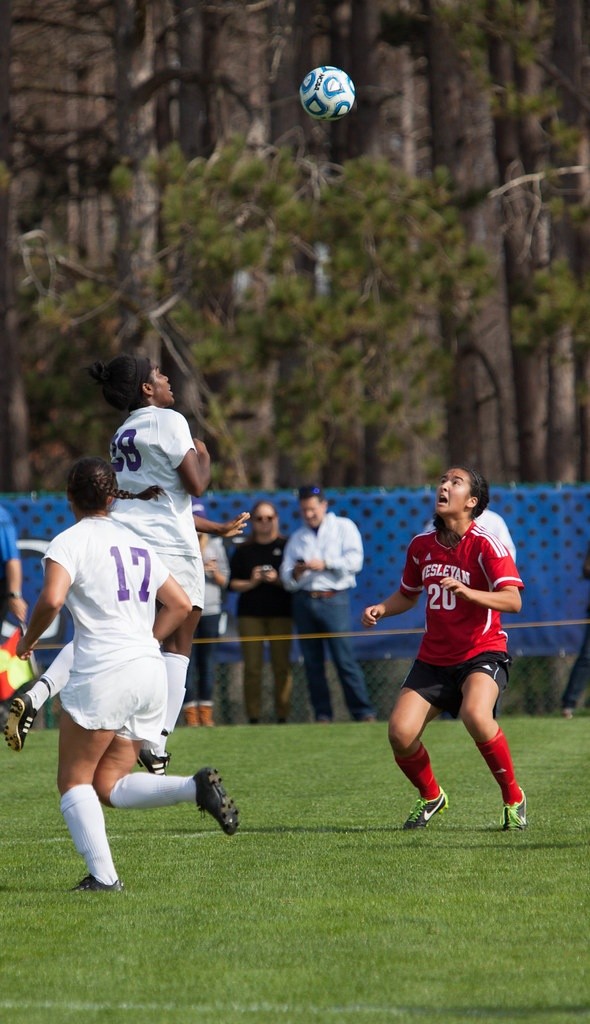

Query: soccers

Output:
300,65,357,121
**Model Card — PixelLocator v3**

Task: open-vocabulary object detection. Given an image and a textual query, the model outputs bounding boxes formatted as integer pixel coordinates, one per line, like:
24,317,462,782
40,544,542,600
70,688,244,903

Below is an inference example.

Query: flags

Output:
0,628,36,702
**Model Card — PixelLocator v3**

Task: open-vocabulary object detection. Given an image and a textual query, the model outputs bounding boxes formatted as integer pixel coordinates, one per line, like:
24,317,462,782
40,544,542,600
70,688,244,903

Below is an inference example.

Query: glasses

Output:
256,516,274,522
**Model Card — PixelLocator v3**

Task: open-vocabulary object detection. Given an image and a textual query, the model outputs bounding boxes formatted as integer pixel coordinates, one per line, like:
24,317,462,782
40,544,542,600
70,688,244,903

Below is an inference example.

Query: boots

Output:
199,701,215,727
184,701,198,726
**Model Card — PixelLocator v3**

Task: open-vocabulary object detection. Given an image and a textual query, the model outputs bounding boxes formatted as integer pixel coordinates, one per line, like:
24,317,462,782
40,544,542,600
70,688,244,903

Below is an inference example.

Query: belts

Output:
307,591,336,599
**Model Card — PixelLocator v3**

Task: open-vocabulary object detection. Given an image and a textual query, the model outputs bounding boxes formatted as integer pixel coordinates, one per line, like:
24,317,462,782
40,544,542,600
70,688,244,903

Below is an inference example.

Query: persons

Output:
560,545,590,718
279,485,376,723
0,505,28,635
423,509,518,719
4,356,251,774
228,502,293,725
361,466,528,832
183,500,231,726
16,459,240,891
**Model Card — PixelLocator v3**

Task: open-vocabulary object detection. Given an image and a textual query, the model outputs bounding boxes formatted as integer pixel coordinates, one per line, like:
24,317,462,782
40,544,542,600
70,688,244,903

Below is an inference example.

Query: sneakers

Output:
404,786,448,828
500,787,527,831
138,748,171,776
69,874,124,893
194,766,240,835
4,694,37,752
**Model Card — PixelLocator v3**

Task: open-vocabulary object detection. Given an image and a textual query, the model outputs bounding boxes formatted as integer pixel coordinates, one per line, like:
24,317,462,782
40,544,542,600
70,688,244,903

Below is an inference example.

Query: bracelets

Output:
9,592,22,597
